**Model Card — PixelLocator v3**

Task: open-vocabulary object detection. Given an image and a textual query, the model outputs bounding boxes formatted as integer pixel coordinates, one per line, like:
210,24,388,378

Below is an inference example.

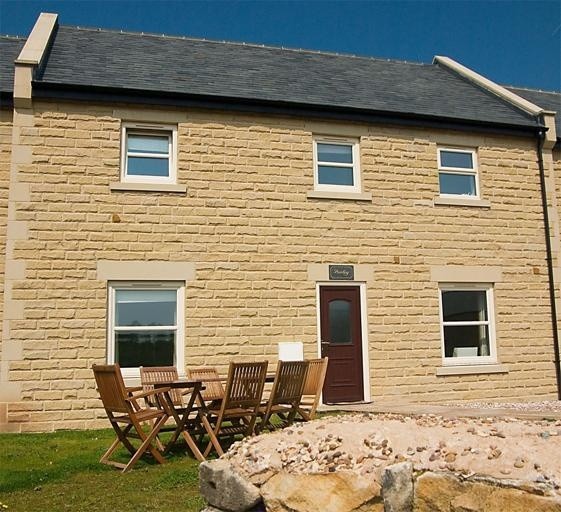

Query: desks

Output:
143,376,276,460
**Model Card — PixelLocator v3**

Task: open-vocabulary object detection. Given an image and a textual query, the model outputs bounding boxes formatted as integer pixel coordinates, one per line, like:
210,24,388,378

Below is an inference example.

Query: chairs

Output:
294,357,330,419
194,360,270,460
92,362,169,474
139,365,198,453
258,359,309,429
186,367,239,443
